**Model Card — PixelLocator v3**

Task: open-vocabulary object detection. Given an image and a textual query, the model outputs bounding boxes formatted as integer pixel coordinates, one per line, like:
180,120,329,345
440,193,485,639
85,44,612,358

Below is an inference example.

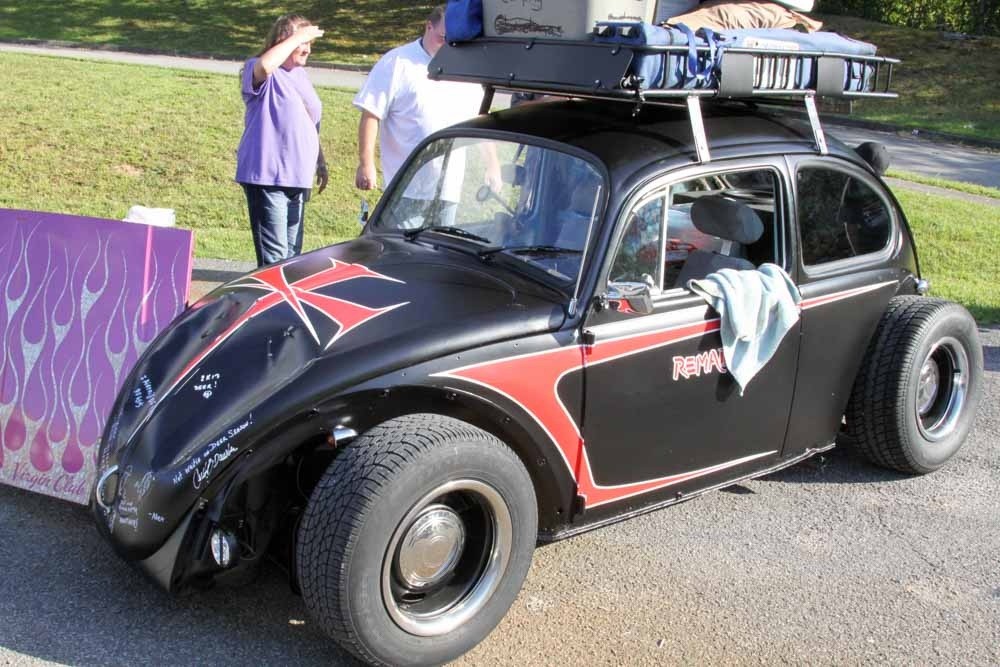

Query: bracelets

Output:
318,163,325,168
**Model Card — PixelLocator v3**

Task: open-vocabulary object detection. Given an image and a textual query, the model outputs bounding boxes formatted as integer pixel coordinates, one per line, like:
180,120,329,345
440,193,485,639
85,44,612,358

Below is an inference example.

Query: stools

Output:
553,178,605,253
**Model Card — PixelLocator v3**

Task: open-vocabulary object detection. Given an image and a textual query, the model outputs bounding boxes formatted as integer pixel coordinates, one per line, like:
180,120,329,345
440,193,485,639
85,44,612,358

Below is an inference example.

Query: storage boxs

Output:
482,0,701,42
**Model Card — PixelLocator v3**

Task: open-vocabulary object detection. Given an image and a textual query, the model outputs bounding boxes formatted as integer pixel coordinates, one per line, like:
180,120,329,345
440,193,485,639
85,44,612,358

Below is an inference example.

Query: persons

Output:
352,4,503,230
235,14,328,266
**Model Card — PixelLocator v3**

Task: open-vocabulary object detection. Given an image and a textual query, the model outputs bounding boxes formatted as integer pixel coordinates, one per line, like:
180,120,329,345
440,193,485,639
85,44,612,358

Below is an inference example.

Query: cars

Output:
88,34,986,667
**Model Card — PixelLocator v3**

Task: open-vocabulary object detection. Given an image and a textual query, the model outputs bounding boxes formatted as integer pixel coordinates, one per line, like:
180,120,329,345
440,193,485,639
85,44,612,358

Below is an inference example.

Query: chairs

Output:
673,195,764,287
